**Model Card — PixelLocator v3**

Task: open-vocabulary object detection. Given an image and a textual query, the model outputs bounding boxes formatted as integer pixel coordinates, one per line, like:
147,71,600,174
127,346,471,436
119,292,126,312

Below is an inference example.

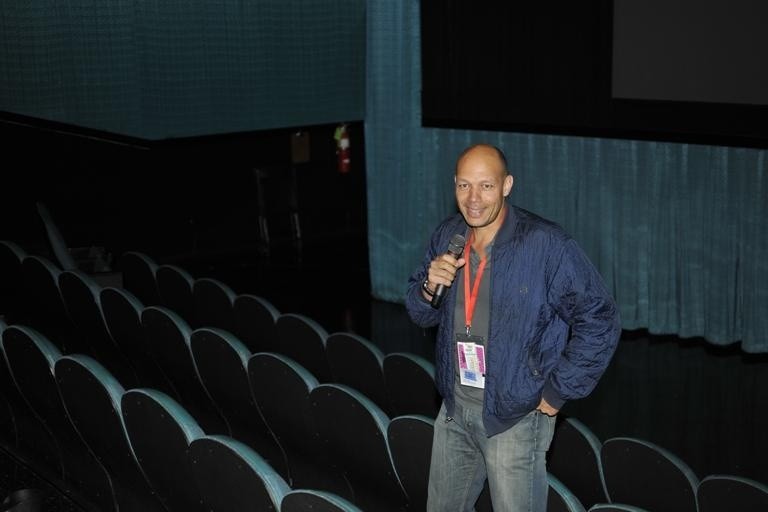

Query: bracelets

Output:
422,279,437,297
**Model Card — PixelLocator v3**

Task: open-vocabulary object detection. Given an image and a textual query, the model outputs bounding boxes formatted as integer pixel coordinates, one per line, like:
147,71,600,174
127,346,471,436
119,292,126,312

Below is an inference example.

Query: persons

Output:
408,144,622,512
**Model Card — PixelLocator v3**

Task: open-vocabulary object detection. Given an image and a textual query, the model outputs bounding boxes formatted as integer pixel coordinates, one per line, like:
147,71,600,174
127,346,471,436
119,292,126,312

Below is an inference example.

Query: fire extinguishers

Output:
335,124,351,173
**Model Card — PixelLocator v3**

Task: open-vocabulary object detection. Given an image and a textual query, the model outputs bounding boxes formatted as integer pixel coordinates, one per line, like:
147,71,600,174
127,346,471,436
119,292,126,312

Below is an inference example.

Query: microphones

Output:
430,235,467,308
